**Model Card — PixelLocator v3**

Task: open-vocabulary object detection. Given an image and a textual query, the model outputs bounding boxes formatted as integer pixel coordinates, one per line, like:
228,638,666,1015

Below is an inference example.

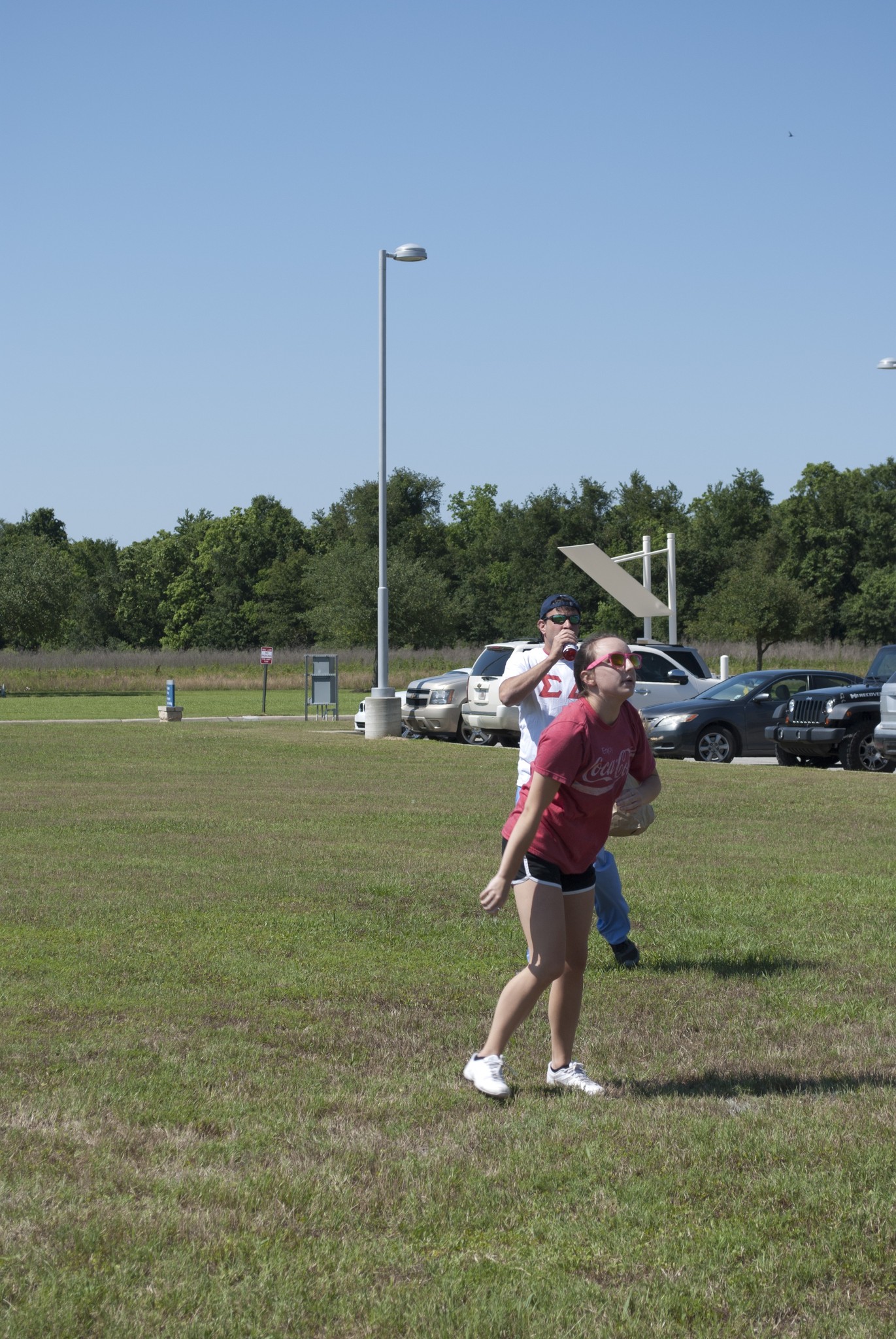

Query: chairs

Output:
773,685,790,699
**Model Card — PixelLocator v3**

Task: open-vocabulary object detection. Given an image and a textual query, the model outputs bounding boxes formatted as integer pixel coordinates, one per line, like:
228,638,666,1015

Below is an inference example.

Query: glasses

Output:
586,652,642,669
543,614,581,625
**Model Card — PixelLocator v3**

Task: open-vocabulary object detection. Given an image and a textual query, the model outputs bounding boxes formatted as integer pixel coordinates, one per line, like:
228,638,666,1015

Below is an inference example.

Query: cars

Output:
638,669,865,763
354,635,713,744
874,670,896,761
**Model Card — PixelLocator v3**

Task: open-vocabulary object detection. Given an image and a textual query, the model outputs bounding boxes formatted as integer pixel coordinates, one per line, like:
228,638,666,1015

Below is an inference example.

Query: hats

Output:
540,594,581,619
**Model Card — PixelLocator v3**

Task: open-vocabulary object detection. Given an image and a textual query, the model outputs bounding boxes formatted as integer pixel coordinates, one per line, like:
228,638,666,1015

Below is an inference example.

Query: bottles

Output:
562,642,577,661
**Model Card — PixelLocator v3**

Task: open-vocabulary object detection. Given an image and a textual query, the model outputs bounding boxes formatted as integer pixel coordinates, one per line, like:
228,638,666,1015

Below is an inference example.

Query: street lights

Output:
378,242,430,687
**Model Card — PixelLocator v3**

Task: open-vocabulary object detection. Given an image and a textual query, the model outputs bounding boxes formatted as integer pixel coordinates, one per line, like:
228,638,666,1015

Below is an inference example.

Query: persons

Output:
498,595,639,968
461,636,662,1098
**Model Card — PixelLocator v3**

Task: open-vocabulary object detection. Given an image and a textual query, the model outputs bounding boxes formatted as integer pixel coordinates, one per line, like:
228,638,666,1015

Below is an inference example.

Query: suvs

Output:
764,644,896,771
461,638,769,749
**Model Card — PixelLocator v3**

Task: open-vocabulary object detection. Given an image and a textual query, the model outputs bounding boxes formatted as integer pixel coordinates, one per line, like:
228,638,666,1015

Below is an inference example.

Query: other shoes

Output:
609,937,640,966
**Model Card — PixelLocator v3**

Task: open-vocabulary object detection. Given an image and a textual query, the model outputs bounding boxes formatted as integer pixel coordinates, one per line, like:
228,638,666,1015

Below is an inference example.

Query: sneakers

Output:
546,1060,605,1094
463,1053,511,1098
